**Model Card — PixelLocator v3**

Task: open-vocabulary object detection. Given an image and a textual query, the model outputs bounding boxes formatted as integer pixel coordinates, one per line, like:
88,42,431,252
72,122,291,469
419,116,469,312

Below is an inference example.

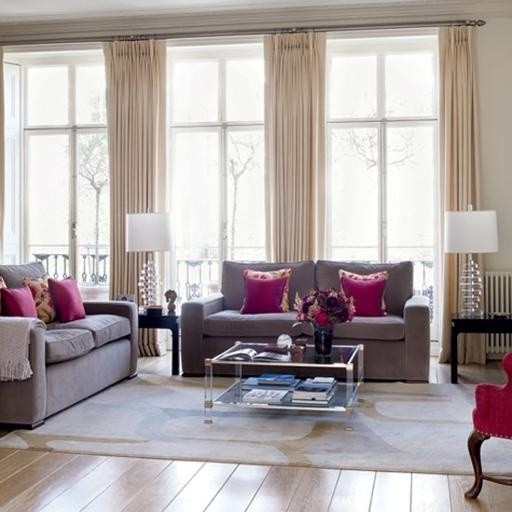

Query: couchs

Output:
1,263,139,430
180,261,431,384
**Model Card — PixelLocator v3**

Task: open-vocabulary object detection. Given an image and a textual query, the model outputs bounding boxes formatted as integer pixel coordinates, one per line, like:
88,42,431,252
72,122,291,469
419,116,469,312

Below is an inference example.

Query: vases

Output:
313,328,333,356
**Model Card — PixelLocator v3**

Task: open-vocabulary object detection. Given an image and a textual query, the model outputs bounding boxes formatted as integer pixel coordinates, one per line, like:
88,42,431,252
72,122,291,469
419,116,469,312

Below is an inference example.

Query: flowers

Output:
284,286,355,331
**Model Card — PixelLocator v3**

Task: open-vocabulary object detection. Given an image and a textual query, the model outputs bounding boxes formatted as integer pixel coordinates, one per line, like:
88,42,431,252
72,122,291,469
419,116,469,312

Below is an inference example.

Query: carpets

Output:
0,373,511,479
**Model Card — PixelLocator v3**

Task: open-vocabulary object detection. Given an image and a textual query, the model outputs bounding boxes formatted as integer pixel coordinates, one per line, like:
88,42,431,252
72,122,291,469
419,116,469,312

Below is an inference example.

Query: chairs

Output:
462,353,512,499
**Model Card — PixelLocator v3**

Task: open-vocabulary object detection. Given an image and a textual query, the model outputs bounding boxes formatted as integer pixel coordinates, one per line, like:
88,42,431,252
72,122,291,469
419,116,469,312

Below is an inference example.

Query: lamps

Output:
123,213,173,309
442,204,501,315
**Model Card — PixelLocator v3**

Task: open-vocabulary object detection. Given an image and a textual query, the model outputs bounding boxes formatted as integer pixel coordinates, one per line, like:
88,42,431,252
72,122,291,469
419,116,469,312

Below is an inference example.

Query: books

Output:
241,373,338,404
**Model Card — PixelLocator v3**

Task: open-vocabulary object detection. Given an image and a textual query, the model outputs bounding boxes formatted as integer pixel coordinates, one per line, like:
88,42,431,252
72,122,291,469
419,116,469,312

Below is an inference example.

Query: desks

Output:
138,313,184,377
448,315,510,385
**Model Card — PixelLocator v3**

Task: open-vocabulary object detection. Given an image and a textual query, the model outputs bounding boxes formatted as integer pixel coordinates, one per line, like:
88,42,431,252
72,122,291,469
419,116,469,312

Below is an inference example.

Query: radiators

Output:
479,269,511,362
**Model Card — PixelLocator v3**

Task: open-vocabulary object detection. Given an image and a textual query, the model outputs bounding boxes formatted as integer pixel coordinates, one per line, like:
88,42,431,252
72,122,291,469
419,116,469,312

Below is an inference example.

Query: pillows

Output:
341,275,386,318
239,266,292,313
1,284,39,318
24,274,58,323
48,276,87,321
242,276,287,314
339,268,389,318
